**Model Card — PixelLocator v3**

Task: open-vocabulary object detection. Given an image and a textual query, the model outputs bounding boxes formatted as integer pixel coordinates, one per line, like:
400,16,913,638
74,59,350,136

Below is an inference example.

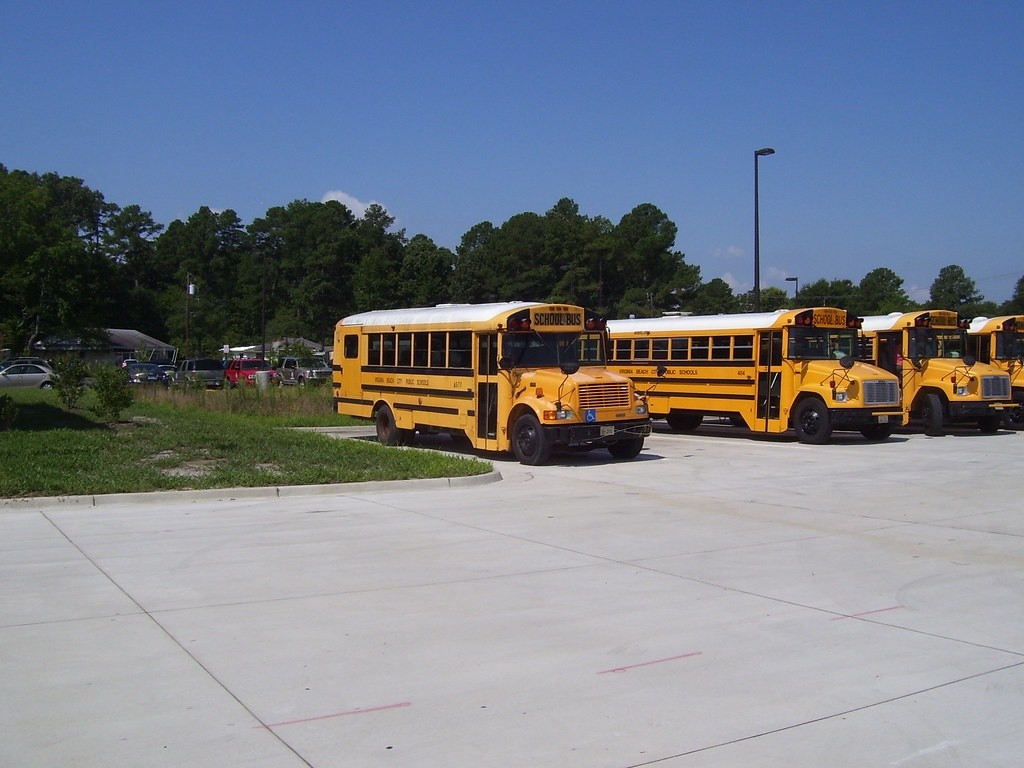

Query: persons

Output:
878,334,902,375
760,338,781,420
1004,337,1015,355
915,335,933,356
538,337,570,367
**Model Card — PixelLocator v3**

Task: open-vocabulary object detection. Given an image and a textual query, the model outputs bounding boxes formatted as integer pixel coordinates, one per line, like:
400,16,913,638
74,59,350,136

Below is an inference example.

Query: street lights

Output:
186,271,197,358
754,148,775,312
786,277,798,309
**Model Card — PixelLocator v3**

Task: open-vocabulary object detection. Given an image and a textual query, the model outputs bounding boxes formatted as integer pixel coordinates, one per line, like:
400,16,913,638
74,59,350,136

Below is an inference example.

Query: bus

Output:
857,310,1021,436
961,315,1024,429
330,301,668,466
605,306,911,445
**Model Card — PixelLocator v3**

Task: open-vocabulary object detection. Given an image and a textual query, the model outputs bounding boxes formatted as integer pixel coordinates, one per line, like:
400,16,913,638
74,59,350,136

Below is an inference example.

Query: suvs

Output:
225,359,280,389
1,357,56,371
170,359,226,389
276,356,332,390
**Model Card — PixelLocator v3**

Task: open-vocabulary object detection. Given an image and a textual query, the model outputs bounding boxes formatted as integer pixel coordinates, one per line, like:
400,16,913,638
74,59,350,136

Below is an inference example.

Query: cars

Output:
0,364,60,389
156,365,178,379
125,364,166,384
